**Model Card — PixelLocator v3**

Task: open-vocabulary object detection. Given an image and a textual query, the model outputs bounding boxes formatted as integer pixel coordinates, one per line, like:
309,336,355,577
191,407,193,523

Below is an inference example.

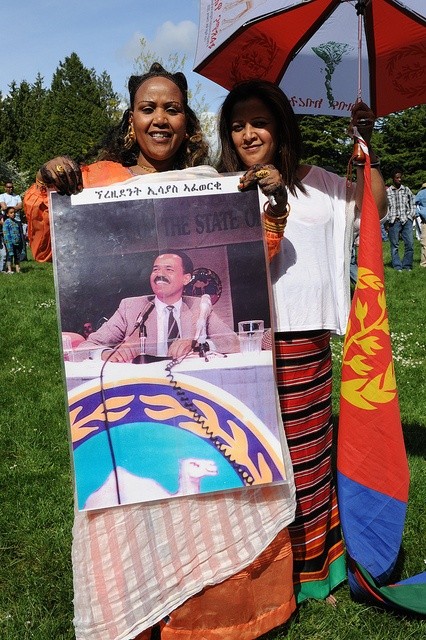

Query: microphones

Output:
190,294,213,348
136,300,154,328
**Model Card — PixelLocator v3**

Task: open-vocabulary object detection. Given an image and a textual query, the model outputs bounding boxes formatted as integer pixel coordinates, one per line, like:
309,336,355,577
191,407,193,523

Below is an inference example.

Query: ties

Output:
166,306,180,350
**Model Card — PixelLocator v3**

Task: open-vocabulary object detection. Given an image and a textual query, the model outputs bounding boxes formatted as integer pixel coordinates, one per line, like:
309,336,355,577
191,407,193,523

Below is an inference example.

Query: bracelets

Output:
350,156,382,169
263,201,291,220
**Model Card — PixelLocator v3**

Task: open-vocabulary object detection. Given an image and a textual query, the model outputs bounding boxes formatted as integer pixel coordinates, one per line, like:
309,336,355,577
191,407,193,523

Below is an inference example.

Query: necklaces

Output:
137,163,157,173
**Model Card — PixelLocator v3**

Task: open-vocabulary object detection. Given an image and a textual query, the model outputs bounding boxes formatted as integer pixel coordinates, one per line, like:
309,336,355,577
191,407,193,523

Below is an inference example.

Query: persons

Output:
0,180,28,261
415,183,426,267
213,78,389,605
0,202,7,274
2,207,23,274
76,251,240,363
22,62,297,640
384,172,417,271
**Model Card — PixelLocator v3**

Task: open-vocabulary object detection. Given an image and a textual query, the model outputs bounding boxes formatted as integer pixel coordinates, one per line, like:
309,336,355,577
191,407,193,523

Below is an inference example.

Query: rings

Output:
255,169,272,178
56,165,63,172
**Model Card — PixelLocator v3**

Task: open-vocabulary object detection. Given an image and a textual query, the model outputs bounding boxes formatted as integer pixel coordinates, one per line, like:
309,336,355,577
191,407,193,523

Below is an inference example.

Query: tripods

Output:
177,320,228,363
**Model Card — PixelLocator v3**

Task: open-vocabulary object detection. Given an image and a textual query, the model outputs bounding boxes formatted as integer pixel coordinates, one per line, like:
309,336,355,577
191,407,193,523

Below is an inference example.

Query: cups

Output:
63,336,74,363
239,320,264,353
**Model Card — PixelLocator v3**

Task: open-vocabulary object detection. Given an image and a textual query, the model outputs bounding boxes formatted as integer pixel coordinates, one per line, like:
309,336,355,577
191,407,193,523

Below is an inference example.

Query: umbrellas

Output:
192,1,426,167
